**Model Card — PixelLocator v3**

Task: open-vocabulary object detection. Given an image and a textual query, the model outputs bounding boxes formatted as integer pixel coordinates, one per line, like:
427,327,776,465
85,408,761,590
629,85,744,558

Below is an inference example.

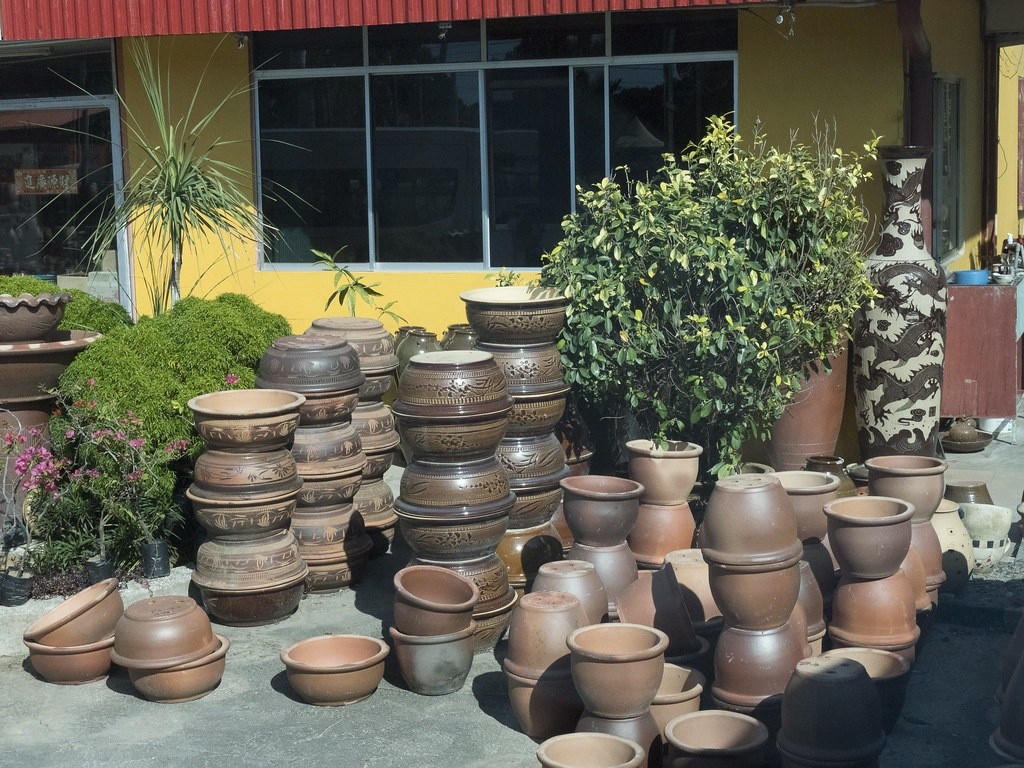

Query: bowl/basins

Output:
991,273,1014,284
23,285,1024,768
952,269,990,285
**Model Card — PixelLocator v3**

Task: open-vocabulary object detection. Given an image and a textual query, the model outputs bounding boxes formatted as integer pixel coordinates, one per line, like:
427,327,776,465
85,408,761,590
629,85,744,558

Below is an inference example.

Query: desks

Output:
940,267,1023,419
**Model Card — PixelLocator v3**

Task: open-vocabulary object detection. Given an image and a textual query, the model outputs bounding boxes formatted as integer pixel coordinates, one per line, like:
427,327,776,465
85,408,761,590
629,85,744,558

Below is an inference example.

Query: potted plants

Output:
0,272,73,342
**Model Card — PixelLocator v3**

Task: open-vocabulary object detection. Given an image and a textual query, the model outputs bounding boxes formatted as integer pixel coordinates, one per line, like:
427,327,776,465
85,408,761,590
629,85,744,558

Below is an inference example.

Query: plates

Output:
937,431,993,454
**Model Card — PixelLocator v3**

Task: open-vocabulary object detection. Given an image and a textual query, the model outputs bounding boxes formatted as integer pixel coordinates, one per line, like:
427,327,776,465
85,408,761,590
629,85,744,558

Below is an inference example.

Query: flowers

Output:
3,395,184,577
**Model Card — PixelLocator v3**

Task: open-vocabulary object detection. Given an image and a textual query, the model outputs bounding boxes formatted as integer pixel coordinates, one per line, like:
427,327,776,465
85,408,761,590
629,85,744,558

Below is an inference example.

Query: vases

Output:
89,552,121,585
1,570,34,608
143,538,169,580
22,578,232,701
849,143,947,463
186,284,1023,767
